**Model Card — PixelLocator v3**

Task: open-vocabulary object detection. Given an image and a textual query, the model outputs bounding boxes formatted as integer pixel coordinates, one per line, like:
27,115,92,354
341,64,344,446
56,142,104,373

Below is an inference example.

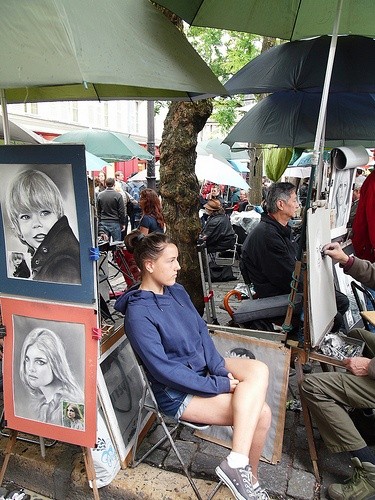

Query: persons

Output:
350,166,375,333
239,181,350,374
299,242,375,500
198,184,253,271
87,171,165,263
9,251,32,279
64,403,85,430
115,231,272,500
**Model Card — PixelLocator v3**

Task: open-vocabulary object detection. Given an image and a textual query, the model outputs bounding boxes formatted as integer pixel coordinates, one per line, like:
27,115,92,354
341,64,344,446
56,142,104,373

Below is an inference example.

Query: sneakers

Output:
328,457,375,500
215,458,256,500
253,482,271,500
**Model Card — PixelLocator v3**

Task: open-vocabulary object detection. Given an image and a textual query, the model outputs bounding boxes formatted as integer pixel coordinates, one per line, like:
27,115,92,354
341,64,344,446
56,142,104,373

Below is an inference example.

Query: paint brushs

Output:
337,350,346,359
320,250,324,254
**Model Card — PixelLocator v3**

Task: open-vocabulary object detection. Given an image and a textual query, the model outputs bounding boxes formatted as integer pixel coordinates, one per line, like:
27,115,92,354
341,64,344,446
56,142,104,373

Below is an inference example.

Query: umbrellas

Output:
154,1,374,156
0,0,235,143
53,128,155,177
128,138,329,190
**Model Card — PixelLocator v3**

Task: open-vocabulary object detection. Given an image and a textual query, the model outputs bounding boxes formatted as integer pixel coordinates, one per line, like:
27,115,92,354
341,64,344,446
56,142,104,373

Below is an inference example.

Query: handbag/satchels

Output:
209,261,232,282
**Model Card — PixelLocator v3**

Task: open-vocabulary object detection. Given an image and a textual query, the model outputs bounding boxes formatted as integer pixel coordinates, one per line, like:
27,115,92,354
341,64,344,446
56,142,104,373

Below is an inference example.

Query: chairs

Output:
129,340,233,500
209,234,241,279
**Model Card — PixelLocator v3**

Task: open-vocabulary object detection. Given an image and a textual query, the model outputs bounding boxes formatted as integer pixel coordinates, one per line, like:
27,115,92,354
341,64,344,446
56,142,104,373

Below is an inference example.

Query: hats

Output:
203,199,221,210
